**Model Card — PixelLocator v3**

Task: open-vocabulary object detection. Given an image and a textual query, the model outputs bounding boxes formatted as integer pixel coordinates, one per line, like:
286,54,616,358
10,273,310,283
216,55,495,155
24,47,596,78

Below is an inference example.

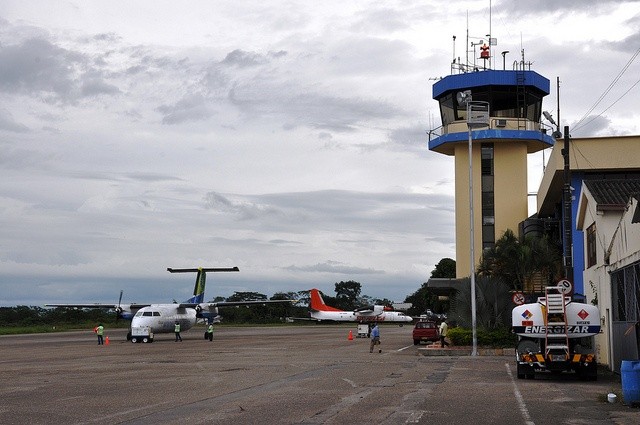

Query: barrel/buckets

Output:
620,360,639,408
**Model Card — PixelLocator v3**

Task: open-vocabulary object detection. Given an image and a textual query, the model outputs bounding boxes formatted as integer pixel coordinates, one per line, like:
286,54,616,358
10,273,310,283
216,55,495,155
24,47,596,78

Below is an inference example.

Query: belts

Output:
375,336,379,337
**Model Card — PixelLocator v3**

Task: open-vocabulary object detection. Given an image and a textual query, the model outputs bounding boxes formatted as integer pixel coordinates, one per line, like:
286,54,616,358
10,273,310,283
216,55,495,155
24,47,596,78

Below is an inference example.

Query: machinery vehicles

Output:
511,287,601,382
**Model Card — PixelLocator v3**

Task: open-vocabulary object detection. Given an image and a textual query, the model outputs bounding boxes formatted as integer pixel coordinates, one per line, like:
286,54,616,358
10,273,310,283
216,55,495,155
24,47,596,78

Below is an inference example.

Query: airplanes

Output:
45,267,299,343
291,288,413,327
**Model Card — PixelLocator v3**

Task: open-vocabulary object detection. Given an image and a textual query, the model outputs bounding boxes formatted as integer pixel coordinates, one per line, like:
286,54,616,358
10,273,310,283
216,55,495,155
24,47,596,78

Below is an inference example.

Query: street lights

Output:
456,89,481,356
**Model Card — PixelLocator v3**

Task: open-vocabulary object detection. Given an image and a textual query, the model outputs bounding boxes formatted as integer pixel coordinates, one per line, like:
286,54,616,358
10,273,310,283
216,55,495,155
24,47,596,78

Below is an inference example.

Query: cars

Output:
413,322,440,345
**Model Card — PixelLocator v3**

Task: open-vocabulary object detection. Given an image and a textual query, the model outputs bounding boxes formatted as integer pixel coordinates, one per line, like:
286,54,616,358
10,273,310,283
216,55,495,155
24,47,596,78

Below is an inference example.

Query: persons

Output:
96,323,104,345
369,323,382,354
174,322,183,342
207,323,214,342
438,317,450,348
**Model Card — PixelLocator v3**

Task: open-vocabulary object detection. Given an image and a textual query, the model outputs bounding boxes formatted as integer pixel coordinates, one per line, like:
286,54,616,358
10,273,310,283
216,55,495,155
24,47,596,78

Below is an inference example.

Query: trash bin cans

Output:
617,358,640,406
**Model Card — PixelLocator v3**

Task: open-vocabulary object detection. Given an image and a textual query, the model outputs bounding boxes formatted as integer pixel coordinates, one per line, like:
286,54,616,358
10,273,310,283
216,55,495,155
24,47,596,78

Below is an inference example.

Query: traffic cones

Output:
347,330,353,340
105,336,110,345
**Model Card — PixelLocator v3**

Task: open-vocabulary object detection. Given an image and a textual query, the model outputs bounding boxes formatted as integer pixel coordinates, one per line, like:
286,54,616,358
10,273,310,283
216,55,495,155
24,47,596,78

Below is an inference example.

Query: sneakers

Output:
379,350,381,353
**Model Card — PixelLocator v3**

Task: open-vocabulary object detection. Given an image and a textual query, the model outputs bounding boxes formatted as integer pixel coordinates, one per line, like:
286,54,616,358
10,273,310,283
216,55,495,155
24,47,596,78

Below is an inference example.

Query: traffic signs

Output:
556,279,573,296
